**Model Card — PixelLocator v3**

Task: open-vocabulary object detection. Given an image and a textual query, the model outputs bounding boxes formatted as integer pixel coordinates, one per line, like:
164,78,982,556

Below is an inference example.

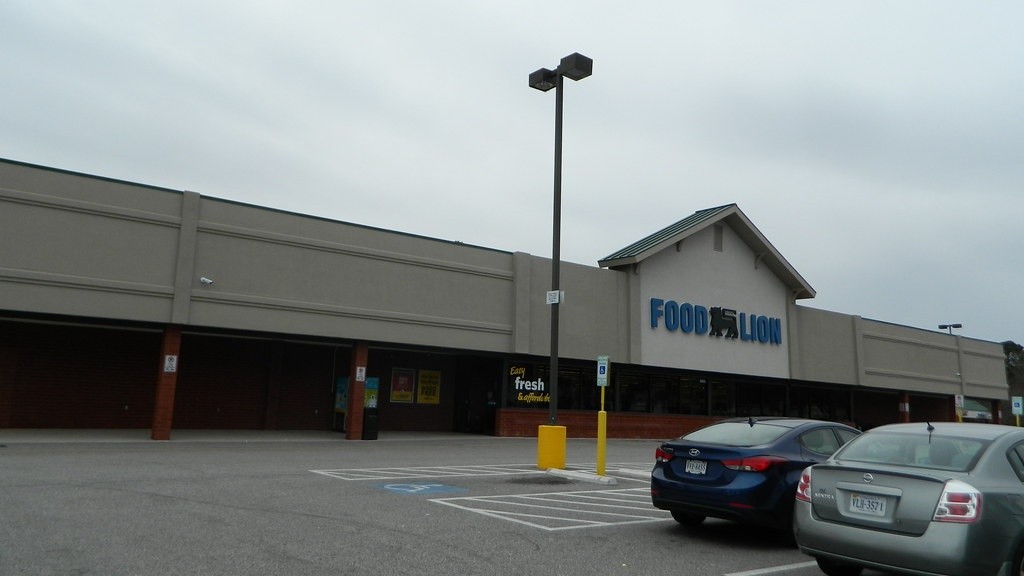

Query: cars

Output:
650,415,862,543
794,421,1024,576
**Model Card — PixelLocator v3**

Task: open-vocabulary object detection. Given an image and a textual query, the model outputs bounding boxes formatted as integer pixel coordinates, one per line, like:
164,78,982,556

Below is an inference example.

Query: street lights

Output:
528,52,593,469
937,324,962,334
